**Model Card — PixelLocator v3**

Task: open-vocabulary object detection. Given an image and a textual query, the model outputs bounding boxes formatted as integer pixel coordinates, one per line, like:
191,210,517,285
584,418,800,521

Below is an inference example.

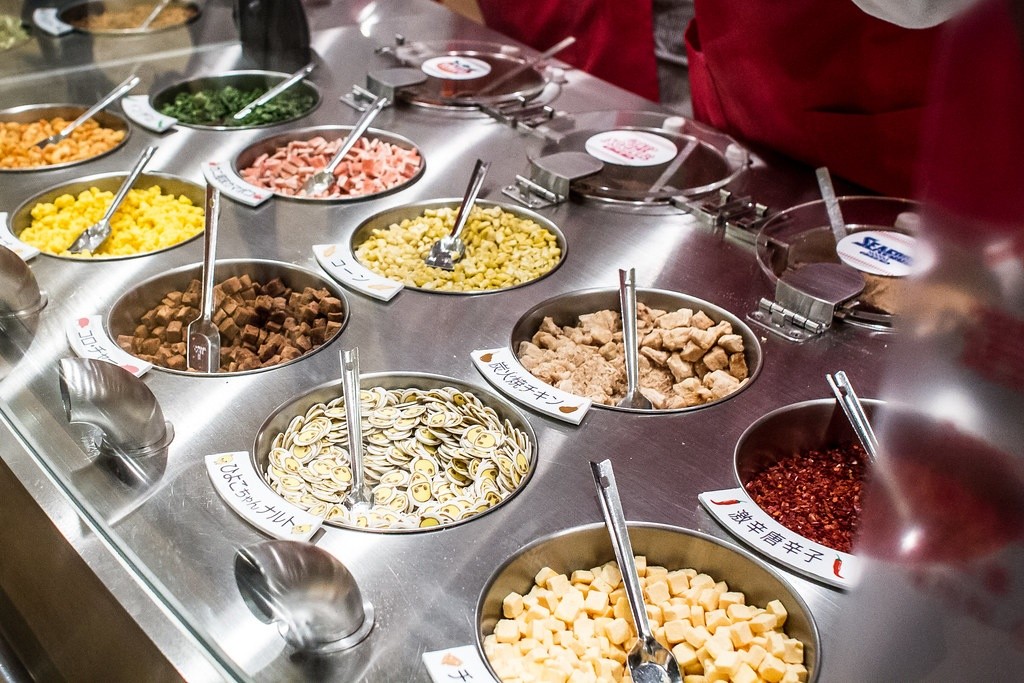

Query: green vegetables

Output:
158,85,314,128
